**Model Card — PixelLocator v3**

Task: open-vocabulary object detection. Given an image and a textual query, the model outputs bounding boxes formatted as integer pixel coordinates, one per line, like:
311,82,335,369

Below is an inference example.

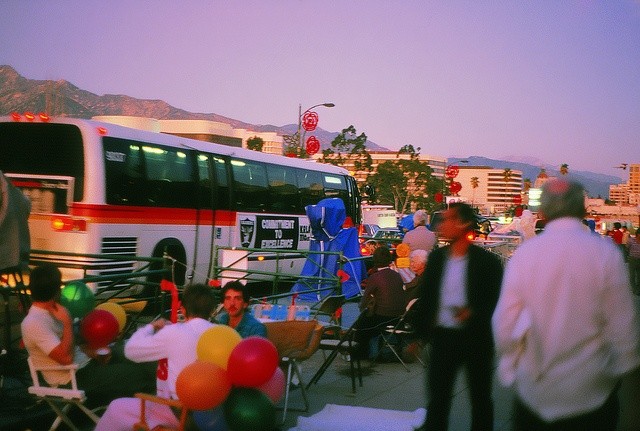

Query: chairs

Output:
131,391,193,430
304,307,368,395
25,356,107,431
373,292,424,374
313,292,346,325
260,320,322,427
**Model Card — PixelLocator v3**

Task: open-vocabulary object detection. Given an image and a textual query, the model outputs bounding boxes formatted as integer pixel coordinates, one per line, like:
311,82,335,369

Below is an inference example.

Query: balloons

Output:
193,405,226,431
80,310,119,346
59,282,95,320
223,388,276,431
259,368,285,405
94,302,127,334
227,337,278,389
197,326,243,372
176,362,232,411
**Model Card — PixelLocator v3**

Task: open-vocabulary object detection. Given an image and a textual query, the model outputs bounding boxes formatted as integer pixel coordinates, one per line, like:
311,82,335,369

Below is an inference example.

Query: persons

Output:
409,202,504,431
21,264,143,404
491,177,640,431
403,209,439,252
403,249,428,300
628,230,640,296
212,280,268,339
621,226,630,263
352,246,405,363
124,283,217,400
608,222,623,250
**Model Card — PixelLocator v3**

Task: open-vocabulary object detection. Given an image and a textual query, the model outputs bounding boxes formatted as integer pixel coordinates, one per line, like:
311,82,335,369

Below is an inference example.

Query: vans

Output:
429,206,494,240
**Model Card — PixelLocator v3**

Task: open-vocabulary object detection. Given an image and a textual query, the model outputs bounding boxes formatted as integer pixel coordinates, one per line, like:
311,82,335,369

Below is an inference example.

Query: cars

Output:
362,227,405,255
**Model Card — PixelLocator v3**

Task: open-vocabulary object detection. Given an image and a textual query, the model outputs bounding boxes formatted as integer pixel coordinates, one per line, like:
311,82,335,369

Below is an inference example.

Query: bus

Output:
0,111,373,301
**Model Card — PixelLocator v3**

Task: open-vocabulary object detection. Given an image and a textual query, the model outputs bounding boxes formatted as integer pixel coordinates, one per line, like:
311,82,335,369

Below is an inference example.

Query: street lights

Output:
295,103,335,158
442,160,468,210
